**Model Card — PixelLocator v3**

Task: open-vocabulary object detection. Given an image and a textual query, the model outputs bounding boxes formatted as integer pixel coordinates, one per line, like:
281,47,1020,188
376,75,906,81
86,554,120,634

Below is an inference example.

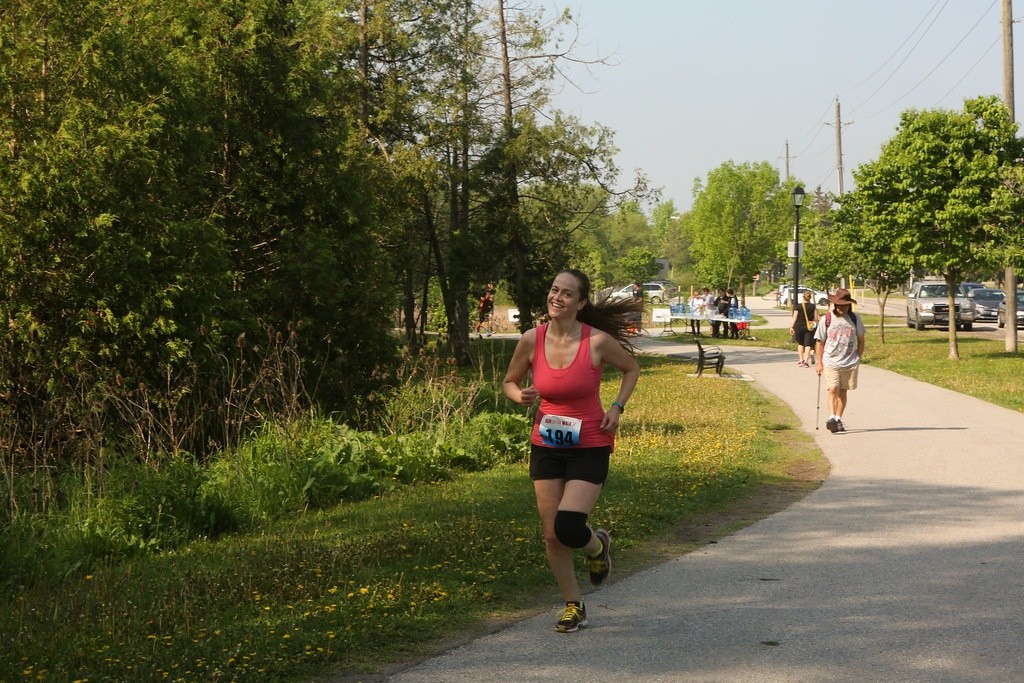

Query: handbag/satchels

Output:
806,321,815,331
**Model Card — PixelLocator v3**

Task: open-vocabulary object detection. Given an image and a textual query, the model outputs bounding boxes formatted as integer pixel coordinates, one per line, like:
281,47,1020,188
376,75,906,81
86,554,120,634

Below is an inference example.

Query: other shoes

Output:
487,333,491,337
475,329,479,333
803,363,809,368
709,333,739,339
836,419,844,431
826,419,838,432
797,361,803,367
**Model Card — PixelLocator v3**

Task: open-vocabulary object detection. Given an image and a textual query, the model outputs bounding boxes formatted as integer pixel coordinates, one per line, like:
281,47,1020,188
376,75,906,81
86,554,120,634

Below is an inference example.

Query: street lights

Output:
791,185,809,317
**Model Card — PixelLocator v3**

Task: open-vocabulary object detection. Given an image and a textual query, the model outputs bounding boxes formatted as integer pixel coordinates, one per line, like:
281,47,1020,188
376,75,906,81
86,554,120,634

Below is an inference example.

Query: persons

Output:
720,289,739,339
689,289,703,335
708,288,729,339
790,290,830,367
476,282,496,337
693,288,715,336
501,268,639,632
814,289,865,433
632,280,643,336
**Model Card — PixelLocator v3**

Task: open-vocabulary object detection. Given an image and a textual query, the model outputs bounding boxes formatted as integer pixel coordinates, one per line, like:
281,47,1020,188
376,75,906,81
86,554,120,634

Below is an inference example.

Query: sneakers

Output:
584,528,612,589
555,599,588,632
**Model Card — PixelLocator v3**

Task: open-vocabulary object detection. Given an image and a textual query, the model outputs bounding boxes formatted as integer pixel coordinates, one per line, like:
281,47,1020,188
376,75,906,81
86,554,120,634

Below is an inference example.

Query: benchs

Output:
694,339,726,378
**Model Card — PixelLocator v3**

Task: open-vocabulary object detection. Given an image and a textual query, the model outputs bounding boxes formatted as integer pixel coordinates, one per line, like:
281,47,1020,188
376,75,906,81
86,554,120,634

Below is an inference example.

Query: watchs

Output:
612,402,624,413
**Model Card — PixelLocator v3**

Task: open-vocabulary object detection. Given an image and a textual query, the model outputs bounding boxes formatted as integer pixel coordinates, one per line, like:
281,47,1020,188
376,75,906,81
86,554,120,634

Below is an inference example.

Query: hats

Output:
830,289,857,305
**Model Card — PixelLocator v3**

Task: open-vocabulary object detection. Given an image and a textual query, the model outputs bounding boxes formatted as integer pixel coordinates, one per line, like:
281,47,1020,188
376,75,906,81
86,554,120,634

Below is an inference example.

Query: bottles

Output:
670,302,677,314
678,303,685,313
728,304,750,320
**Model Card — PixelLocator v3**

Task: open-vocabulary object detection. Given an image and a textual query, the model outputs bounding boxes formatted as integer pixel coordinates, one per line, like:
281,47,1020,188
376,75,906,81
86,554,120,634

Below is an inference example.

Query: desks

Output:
710,318,758,341
657,313,706,340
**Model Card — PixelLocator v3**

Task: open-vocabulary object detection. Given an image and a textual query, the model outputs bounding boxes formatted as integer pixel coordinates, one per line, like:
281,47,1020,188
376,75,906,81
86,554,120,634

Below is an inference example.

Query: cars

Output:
996,291,1024,328
961,282,988,294
966,288,1006,322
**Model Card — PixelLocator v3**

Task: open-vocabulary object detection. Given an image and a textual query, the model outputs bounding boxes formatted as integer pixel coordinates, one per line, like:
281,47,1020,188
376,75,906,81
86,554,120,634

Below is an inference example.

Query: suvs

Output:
905,279,976,331
609,280,669,306
778,287,832,306
